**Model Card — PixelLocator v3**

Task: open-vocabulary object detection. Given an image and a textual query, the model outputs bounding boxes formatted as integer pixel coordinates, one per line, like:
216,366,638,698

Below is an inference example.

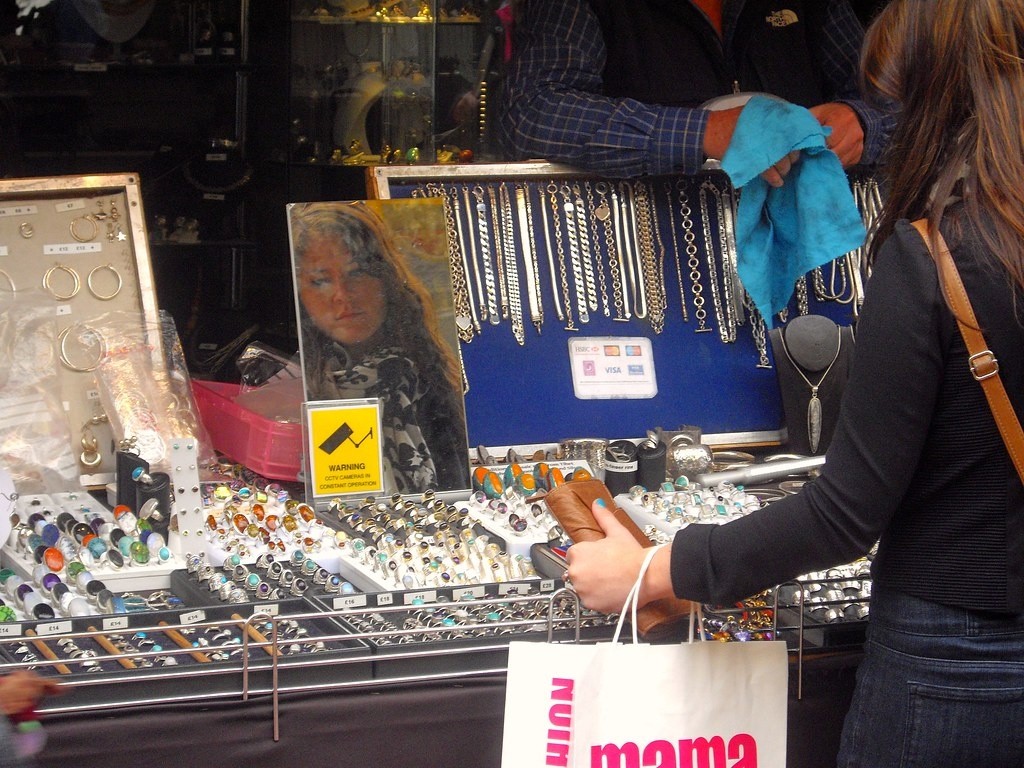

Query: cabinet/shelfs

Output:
286,14,490,168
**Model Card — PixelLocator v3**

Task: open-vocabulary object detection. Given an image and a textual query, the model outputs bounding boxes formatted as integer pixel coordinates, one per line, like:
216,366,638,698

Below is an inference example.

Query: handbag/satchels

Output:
501,542,788,768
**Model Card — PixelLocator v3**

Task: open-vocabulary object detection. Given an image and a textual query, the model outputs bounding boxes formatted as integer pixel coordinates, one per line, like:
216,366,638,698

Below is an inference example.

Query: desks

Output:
12,641,867,768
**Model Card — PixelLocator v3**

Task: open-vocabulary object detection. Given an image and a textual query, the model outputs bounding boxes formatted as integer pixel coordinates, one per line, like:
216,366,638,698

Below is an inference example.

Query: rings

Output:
563,570,571,585
330,140,419,166
469,431,769,545
0,435,628,673
792,560,874,622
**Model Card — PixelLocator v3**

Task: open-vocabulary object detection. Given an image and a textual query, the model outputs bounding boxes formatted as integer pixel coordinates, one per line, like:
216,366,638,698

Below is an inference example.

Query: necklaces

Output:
341,27,372,62
350,5,372,14
347,73,367,103
777,325,841,454
183,156,252,193
393,26,418,52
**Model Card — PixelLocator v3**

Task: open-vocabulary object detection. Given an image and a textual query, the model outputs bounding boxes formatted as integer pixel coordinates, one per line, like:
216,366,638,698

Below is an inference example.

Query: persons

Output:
290,201,469,497
490,0,900,187
563,0,1024,768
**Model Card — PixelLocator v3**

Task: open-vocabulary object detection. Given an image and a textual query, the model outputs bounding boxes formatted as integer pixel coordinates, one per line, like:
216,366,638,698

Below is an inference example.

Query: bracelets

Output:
412,177,886,369
